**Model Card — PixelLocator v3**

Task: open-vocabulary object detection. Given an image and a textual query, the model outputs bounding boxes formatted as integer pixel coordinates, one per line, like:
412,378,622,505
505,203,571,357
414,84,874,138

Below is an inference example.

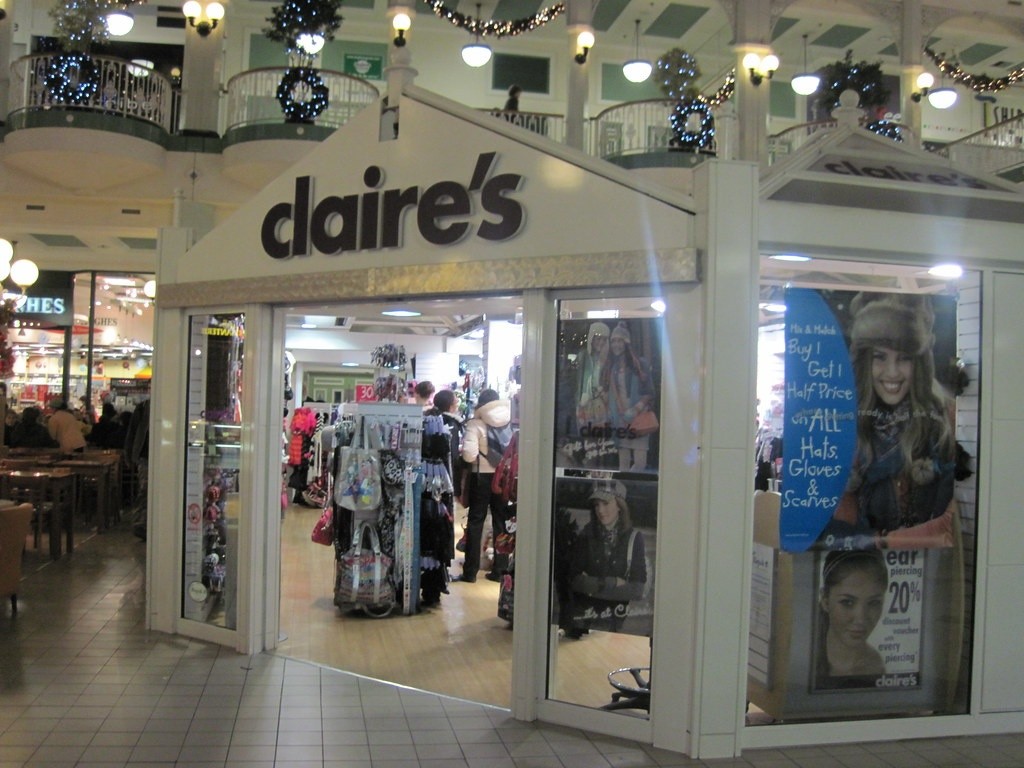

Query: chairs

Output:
0,502,32,616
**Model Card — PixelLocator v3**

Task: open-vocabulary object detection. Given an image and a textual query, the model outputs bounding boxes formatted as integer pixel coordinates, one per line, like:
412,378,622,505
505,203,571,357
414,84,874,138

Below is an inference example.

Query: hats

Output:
849,291,938,356
609,321,631,344
587,322,610,354
588,480,626,501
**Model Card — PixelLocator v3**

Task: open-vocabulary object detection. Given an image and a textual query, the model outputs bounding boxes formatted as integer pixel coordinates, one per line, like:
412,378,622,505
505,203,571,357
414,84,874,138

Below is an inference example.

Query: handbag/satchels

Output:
625,530,652,600
310,413,396,609
629,408,659,435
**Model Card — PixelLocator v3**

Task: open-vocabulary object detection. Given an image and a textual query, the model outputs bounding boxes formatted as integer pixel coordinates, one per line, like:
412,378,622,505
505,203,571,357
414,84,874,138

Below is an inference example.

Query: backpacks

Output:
477,417,513,467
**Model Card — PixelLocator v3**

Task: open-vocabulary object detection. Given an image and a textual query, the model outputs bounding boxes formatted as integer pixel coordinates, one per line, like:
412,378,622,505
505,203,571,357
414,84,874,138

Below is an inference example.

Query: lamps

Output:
460,2,492,69
143,279,156,306
102,7,136,35
183,1,226,38
127,43,155,77
293,31,326,55
622,19,652,83
928,70,958,110
573,31,595,65
909,73,934,103
392,12,411,48
790,34,820,95
838,88,860,108
743,52,779,87
0,236,39,310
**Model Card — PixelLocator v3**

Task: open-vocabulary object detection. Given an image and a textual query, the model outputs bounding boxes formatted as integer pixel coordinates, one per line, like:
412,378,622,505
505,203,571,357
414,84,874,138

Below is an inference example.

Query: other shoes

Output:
458,574,476,582
486,573,502,582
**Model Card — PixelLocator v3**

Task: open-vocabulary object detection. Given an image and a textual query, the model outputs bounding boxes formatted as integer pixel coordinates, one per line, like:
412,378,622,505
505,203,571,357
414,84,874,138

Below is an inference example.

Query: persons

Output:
818,547,891,677
566,480,646,631
415,382,434,405
4,379,151,538
867,107,901,141
455,389,515,583
566,320,657,469
831,291,957,544
426,391,462,488
501,84,520,121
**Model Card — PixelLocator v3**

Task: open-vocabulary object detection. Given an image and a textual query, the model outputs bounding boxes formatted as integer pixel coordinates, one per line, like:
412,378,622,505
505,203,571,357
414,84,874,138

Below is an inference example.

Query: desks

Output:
1,445,121,560
